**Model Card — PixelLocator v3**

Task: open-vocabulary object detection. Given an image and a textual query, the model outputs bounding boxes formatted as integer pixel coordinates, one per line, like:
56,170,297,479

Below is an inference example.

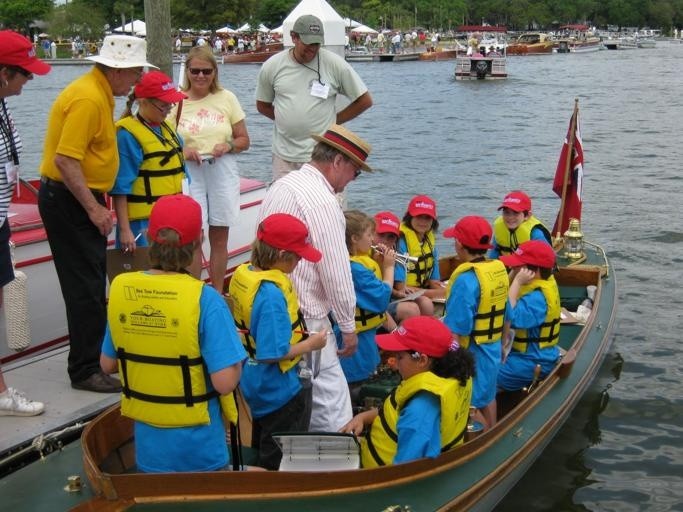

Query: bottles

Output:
564,217,583,259
465,406,485,443
297,360,314,389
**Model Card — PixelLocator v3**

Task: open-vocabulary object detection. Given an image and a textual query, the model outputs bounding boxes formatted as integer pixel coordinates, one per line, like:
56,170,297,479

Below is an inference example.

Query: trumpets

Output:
370,244,419,266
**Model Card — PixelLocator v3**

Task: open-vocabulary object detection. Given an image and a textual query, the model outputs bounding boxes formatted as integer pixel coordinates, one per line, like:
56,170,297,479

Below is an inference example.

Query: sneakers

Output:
70,371,122,392
0,387,45,417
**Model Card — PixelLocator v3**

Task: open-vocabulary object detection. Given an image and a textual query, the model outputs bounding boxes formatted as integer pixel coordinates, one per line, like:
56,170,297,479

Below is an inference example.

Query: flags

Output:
550,110,586,238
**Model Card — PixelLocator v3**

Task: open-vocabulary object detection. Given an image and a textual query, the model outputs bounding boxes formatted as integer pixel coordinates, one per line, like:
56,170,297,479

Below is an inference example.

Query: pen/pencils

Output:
293,330,333,335
122,233,141,255
236,329,248,334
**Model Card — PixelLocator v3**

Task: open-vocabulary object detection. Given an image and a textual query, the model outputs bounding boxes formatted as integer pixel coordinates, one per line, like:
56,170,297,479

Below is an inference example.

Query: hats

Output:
498,191,531,214
293,15,325,44
147,193,203,246
134,70,189,104
83,33,163,72
444,215,494,251
311,123,375,173
375,314,454,360
408,195,436,221
372,211,400,237
257,213,323,263
499,239,556,269
0,28,52,76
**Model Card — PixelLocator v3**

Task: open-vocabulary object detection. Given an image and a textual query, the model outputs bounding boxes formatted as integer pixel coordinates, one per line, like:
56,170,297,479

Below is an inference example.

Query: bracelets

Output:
225,140,235,153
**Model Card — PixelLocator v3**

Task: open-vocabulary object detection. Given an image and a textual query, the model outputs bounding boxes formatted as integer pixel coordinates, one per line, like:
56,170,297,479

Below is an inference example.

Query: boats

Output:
64,231,616,512
486,350,624,512
1,167,273,372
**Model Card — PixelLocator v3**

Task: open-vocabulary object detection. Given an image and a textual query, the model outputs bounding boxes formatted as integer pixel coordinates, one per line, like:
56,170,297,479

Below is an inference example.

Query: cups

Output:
586,285,597,301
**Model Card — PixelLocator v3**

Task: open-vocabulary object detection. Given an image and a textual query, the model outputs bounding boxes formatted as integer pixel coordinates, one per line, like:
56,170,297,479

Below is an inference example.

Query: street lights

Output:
129,5,135,36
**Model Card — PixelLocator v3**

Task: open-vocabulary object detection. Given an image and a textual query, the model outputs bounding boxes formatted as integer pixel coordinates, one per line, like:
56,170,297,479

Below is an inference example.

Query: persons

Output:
0,27,47,419
254,12,373,183
95,190,251,474
36,33,162,392
161,42,251,297
224,211,327,473
259,121,374,436
96,70,193,375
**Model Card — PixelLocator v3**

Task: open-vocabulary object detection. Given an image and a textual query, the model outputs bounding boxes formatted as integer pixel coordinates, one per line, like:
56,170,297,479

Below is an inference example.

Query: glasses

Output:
187,66,217,75
13,67,32,79
148,100,176,114
346,158,361,177
